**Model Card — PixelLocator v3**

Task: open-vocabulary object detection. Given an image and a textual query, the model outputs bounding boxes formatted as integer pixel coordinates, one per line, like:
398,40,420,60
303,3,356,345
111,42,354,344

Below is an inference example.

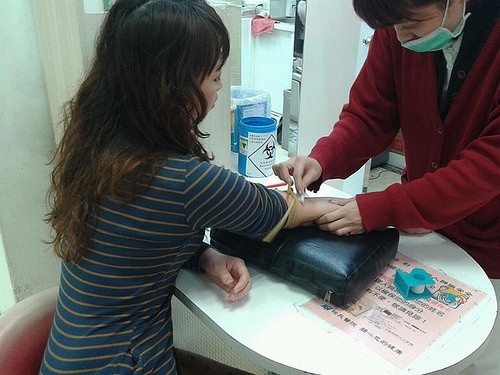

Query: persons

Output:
38,1,433,375
272,0,500,279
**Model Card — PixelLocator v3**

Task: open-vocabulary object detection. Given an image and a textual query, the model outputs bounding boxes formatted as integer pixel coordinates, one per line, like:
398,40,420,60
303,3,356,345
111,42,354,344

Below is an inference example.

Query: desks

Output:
173,184,498,375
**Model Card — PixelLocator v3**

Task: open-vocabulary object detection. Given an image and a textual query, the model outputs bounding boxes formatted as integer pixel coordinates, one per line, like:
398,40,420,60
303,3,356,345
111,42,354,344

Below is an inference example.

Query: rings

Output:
348,231,352,236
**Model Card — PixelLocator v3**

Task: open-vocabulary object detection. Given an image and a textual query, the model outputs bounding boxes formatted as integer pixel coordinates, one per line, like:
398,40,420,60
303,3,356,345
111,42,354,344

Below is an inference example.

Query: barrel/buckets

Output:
238,116,277,178
